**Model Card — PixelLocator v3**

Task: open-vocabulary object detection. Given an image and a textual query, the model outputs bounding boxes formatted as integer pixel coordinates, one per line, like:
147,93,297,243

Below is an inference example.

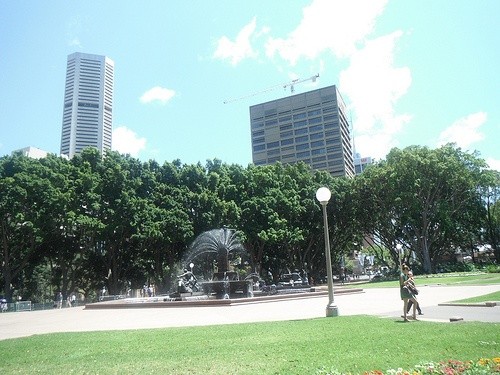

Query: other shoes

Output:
419,313,423,315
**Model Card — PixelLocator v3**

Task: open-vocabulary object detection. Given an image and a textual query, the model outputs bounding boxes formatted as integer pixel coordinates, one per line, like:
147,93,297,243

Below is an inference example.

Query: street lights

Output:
316,187,339,317
396,243,404,289
189,262,194,281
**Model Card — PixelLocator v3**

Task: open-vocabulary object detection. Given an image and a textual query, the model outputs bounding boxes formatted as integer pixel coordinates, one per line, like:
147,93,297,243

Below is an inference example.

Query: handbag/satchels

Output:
406,280,418,295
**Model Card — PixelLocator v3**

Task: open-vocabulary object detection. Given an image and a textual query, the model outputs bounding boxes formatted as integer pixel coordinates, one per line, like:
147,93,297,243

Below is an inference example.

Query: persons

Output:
399,264,424,322
57,291,63,309
140,288,152,297
177,269,199,292
352,273,359,278
67,292,76,307
